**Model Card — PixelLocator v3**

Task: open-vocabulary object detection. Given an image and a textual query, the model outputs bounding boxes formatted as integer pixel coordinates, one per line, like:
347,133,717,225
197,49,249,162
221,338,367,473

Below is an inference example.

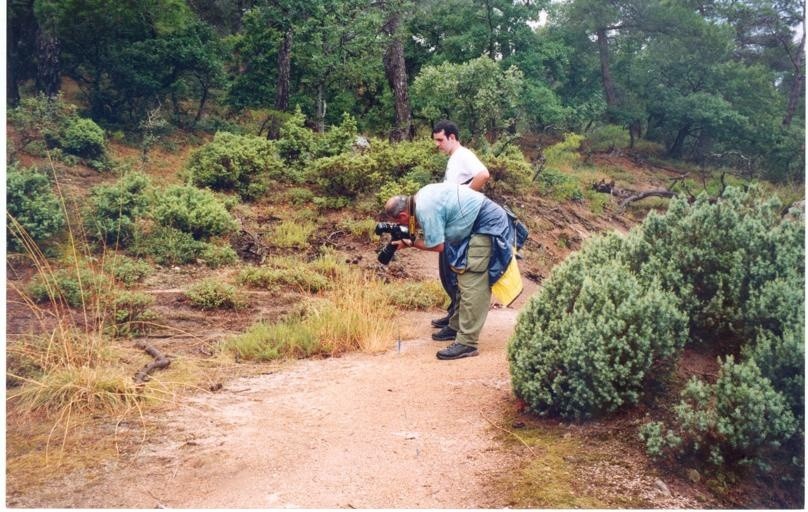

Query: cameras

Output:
375,223,416,266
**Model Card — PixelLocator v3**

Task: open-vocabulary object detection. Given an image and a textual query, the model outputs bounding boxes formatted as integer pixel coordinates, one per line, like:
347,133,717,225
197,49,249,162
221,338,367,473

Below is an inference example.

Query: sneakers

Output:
436,342,480,360
431,328,457,341
431,316,450,327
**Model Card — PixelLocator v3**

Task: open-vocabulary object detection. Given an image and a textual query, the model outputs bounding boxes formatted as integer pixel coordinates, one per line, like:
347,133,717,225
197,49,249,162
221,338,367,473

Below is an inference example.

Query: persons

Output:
428,118,491,328
384,183,508,361
